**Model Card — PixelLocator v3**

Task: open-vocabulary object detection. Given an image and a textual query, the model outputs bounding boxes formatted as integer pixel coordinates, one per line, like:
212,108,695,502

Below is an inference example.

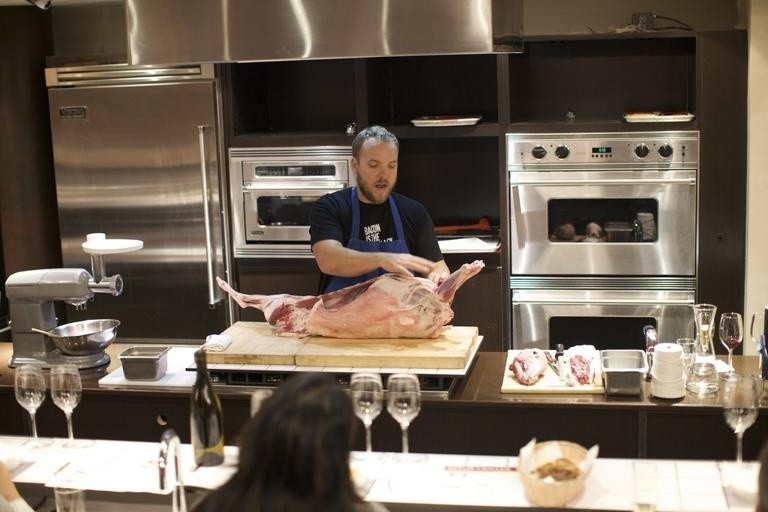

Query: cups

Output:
676,338,695,368
50,484,88,512
249,388,274,417
648,342,687,401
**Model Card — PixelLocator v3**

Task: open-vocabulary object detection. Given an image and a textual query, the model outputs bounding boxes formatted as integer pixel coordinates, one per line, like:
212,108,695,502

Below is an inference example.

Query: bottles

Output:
685,301,719,394
185,348,227,470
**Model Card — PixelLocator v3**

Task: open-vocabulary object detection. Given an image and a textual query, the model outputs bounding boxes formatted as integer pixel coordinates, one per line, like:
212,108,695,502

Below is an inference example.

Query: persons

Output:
190,371,393,512
306,124,451,294
0,461,33,512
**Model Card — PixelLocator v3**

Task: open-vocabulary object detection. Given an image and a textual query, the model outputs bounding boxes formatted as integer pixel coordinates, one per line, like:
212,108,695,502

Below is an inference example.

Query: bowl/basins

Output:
48,317,120,355
516,440,594,508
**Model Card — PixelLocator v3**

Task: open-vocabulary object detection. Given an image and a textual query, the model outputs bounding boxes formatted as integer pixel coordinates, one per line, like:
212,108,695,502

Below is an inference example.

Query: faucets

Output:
156,427,188,512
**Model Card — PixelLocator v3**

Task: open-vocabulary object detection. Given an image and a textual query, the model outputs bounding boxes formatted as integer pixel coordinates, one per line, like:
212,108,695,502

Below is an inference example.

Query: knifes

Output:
554,343,568,382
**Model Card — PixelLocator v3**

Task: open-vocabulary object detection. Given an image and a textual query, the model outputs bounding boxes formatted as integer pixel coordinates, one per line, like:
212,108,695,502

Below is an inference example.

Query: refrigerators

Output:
48,80,230,341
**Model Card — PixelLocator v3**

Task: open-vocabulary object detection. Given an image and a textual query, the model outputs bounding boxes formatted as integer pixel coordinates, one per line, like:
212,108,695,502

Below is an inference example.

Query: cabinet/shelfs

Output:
235,257,512,351
219,29,700,131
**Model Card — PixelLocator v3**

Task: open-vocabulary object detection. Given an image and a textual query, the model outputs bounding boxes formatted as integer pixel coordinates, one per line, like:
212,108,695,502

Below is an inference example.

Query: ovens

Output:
504,129,700,351
228,149,358,259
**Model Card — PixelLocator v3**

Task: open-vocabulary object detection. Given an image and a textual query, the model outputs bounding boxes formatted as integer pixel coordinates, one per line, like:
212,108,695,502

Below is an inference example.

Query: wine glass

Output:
49,363,82,441
13,363,47,440
385,374,421,456
348,371,385,459
721,371,758,463
717,311,743,373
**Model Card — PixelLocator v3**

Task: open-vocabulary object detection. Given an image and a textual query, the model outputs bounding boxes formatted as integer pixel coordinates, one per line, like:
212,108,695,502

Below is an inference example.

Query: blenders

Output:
5,231,145,378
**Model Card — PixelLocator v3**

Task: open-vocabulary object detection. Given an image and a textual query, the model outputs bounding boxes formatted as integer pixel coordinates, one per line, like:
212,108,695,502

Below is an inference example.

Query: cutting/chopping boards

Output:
498,347,603,396
200,320,481,371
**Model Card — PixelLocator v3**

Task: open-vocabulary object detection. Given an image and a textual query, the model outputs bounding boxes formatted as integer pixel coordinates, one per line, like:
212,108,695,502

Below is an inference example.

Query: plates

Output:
410,115,483,130
620,110,694,122
